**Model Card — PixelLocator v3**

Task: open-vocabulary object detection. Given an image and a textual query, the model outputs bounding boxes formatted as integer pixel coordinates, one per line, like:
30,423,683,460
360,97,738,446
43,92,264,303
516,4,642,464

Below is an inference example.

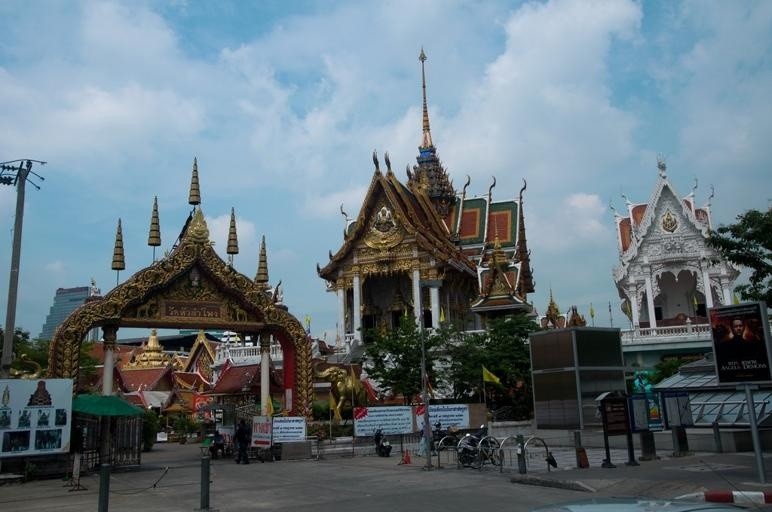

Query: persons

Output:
232,418,252,464
716,316,769,381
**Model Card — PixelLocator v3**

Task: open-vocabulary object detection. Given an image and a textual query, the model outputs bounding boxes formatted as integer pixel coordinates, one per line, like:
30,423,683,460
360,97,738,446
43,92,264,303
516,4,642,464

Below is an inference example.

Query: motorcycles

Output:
371,427,393,458
458,422,503,472
431,421,461,451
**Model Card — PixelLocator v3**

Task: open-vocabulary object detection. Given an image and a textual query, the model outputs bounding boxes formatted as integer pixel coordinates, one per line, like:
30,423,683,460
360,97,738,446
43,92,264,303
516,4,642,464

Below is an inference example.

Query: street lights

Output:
0,158,49,378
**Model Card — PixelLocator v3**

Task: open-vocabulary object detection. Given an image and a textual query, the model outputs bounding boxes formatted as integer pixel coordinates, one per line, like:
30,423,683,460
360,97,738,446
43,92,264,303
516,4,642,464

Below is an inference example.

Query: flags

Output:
350,365,360,395
732,294,739,303
439,306,446,323
402,305,408,320
692,296,698,311
608,301,611,313
625,301,632,318
589,302,595,317
482,365,500,385
329,392,343,421
267,394,273,416
